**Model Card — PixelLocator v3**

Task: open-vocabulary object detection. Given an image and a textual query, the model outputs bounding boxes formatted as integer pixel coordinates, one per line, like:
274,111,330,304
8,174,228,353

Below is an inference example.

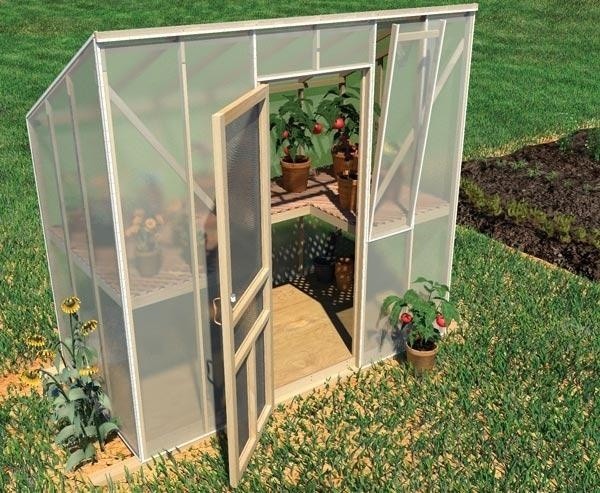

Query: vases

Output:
336,170,358,211
335,254,354,292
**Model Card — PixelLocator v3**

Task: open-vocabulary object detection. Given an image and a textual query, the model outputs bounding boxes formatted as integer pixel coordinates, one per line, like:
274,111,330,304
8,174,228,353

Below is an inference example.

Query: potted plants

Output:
126,208,163,276
313,86,382,179
379,277,459,370
268,93,323,193
